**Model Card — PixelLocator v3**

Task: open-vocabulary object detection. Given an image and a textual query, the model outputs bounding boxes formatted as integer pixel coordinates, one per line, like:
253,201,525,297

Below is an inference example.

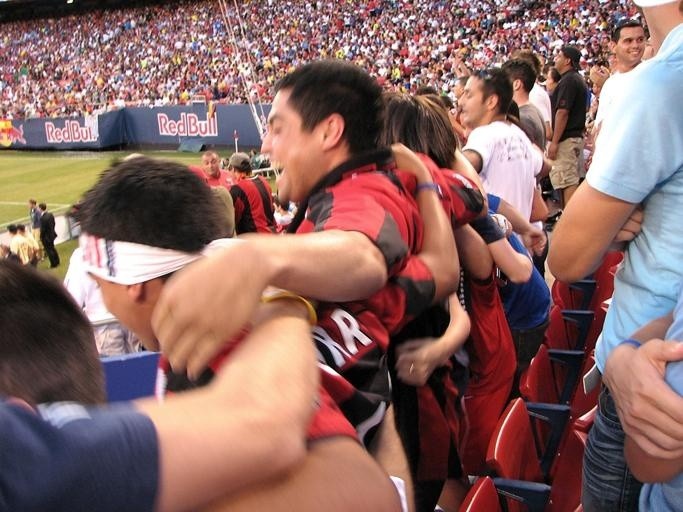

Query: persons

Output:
0,1,655,122
541,2,683,512
0,62,548,512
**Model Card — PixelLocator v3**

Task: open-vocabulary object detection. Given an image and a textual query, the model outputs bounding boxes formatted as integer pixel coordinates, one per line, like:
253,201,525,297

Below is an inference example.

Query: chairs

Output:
457,251,626,511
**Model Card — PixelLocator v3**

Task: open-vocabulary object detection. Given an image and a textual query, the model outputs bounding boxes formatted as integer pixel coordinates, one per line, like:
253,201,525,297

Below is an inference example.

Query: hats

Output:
224,153,249,168
